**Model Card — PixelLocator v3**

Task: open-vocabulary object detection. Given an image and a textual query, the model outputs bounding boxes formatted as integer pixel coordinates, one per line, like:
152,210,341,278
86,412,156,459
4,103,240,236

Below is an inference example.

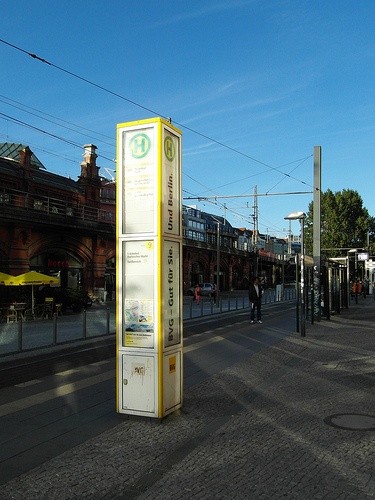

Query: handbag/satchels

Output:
197,291,201,296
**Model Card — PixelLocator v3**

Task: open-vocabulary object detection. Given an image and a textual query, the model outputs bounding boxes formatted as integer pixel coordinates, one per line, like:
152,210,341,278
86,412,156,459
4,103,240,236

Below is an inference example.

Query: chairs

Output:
6,305,18,323
23,307,38,321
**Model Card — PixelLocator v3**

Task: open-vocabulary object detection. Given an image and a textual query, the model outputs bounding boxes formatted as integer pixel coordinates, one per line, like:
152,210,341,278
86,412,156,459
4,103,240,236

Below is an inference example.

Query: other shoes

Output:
251,320,254,324
257,320,263,324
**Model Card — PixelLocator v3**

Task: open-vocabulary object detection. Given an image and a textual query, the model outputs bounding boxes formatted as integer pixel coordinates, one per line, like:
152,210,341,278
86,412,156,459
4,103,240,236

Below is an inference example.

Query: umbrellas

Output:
0,270,61,312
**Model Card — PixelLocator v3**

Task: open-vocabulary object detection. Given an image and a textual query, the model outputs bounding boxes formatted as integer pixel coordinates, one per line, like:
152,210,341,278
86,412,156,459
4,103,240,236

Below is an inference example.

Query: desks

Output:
4,301,28,321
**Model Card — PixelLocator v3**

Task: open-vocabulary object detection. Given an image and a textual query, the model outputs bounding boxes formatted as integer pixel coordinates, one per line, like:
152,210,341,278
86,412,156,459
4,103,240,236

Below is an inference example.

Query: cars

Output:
35,287,93,312
185,283,216,296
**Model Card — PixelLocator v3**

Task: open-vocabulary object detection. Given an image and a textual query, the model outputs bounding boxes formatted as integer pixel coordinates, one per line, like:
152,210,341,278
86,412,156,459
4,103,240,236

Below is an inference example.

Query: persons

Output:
193,284,201,304
249,277,263,323
209,284,217,305
